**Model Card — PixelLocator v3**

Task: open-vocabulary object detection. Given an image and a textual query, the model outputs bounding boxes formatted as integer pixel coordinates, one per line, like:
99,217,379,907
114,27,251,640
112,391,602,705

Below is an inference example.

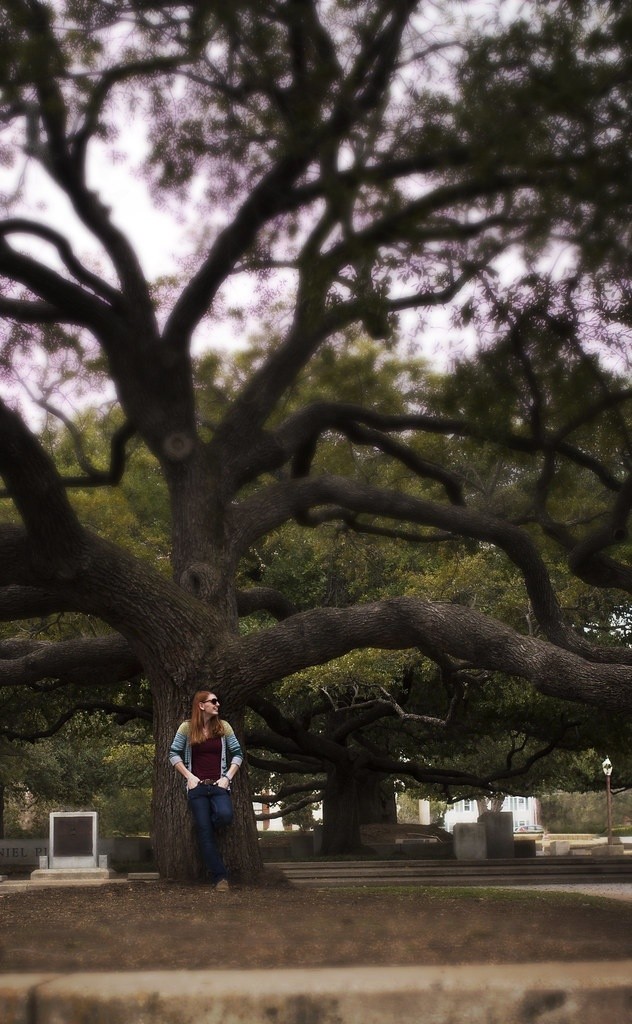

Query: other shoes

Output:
216,879,229,892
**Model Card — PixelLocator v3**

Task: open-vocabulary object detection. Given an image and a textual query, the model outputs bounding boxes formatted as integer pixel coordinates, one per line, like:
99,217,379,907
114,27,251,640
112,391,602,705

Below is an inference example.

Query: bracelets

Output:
223,775,232,783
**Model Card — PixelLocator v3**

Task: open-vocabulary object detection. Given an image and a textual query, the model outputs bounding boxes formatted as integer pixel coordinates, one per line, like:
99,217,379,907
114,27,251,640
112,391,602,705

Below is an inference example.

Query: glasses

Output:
201,698,219,704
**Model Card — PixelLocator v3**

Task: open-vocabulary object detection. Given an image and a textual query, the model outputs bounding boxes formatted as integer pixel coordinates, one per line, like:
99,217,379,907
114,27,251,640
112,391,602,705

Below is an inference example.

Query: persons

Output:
168,690,243,894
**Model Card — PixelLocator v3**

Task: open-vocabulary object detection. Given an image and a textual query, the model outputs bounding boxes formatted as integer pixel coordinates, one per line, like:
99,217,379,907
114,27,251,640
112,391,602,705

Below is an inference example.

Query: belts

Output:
198,778,217,785
